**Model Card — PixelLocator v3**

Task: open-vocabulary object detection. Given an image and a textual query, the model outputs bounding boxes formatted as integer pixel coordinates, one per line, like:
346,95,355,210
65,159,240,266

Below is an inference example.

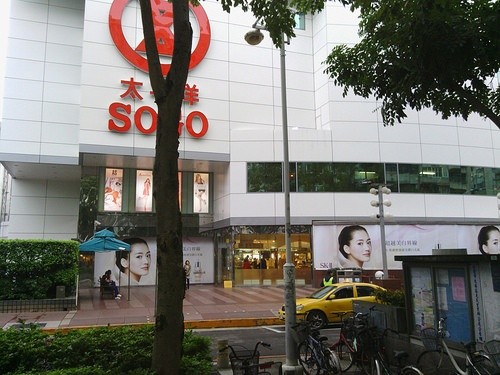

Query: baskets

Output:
229,349,260,375
291,324,308,343
482,340,500,373
419,327,437,351
258,361,282,375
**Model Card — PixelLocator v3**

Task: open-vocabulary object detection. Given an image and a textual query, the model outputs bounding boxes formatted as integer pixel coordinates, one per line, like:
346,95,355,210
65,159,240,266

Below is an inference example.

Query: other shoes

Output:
115,296,120,300
117,294,121,297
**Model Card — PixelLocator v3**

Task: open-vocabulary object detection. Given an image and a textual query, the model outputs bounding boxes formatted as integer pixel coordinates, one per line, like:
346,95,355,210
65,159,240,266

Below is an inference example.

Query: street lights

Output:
369,185,392,280
244,5,305,375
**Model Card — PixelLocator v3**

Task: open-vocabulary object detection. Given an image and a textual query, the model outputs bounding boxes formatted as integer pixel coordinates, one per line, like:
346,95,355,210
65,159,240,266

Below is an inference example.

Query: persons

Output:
339,225,372,268
101,270,123,300
115,238,151,285
184,261,191,289
240,255,308,270
478,225,500,254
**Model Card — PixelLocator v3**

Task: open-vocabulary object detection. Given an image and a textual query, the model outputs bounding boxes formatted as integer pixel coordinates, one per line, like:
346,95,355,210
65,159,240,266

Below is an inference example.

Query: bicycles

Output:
219,341,282,375
416,316,500,375
288,319,342,375
330,306,423,375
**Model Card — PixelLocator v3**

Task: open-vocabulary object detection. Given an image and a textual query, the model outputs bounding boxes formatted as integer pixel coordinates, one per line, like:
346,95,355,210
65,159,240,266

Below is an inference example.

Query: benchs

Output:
99,277,115,298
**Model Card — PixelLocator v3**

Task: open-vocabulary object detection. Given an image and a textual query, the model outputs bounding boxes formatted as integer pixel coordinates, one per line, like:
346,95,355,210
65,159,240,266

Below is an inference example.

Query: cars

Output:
278,280,390,330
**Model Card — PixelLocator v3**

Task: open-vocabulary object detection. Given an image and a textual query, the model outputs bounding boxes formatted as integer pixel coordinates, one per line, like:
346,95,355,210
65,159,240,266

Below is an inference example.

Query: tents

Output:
75,228,132,301
